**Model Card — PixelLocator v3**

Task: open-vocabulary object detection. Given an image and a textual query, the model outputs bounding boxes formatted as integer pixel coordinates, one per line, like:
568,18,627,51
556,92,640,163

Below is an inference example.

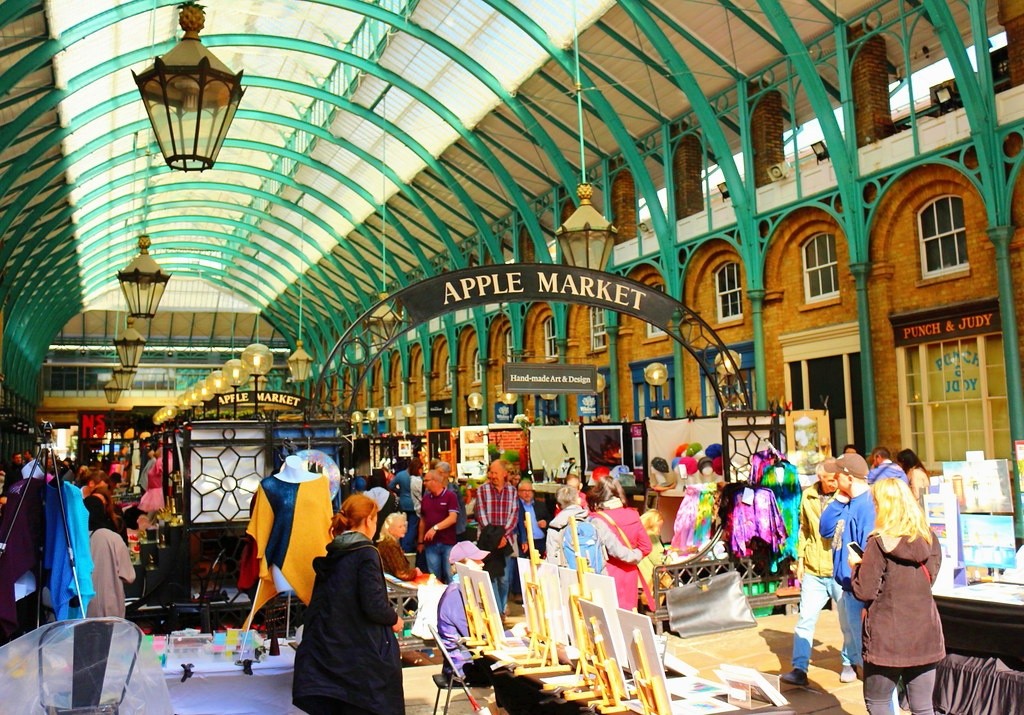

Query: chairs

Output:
37,619,143,714
427,624,596,715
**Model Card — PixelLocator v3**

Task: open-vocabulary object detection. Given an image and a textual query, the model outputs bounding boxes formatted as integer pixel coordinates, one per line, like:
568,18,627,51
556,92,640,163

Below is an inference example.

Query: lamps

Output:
102,367,137,403
645,362,667,386
812,141,829,164
116,235,174,321
351,403,413,433
715,350,740,375
933,86,955,115
717,182,729,202
597,373,605,394
151,345,274,426
362,91,404,353
245,210,269,392
130,0,248,173
553,0,622,273
286,191,316,382
468,391,556,408
113,315,146,368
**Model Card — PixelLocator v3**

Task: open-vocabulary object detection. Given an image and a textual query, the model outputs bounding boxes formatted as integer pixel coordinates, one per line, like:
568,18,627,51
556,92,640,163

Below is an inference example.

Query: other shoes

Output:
841,665,858,682
780,668,809,685
403,547,417,556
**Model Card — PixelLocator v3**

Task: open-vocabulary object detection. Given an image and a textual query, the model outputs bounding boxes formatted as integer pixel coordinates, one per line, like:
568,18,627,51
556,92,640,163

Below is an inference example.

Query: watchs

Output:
433,524,440,533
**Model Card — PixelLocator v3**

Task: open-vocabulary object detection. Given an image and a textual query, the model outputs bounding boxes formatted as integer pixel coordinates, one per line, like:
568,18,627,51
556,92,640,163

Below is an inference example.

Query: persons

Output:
291,494,408,715
509,464,654,613
141,446,174,508
847,476,942,715
436,540,544,715
474,458,519,622
842,444,932,509
781,457,859,687
350,455,478,657
44,453,123,532
83,510,136,620
245,455,334,607
0,448,50,515
819,452,899,715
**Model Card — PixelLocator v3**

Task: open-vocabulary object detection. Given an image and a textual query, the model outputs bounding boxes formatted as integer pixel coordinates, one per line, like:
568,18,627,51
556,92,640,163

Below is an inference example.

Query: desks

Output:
124,576,418,641
927,581,1024,660
55,637,309,715
463,640,842,715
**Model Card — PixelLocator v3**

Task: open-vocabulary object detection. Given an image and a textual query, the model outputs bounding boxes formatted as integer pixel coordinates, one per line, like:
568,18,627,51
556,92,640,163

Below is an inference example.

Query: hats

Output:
679,457,698,475
698,457,713,474
824,453,869,479
363,487,389,512
685,442,702,457
675,442,689,457
710,457,722,476
652,457,669,473
109,472,122,483
449,541,491,564
672,457,680,471
705,443,722,458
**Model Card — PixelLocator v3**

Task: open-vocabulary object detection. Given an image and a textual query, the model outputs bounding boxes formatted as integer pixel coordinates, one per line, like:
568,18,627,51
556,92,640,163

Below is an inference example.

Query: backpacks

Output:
564,520,605,573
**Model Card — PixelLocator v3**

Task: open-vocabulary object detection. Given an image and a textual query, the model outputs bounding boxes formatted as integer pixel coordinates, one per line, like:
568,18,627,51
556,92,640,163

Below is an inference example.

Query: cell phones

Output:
848,542,864,559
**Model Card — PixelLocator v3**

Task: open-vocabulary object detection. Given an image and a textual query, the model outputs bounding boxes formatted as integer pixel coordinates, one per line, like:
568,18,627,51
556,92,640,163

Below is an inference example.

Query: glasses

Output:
519,490,535,492
423,479,430,482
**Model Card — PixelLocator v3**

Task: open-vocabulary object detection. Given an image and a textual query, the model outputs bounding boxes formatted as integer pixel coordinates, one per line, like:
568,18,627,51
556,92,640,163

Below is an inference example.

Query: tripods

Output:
0,422,86,629
